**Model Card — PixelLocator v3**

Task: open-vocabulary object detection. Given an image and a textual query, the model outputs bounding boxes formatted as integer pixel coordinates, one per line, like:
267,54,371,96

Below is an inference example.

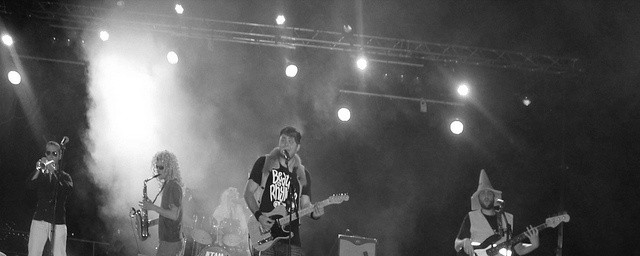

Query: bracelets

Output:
310,212,320,220
254,210,263,221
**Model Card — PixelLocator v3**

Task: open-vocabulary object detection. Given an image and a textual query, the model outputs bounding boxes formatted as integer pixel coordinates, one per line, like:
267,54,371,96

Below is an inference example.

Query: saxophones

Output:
129,173,162,240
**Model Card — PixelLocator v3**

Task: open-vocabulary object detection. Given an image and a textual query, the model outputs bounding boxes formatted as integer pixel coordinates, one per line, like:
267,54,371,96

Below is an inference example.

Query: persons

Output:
244,126,325,256
212,186,248,249
138,151,185,256
24,141,73,256
455,169,539,256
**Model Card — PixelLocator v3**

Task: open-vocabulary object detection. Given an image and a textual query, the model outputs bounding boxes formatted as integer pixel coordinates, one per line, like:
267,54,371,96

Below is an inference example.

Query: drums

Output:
189,215,217,244
219,218,242,247
197,244,231,256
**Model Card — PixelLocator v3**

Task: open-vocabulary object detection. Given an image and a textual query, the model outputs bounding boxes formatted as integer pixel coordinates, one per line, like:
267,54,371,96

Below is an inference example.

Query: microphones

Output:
283,149,291,160
495,198,505,204
61,137,69,145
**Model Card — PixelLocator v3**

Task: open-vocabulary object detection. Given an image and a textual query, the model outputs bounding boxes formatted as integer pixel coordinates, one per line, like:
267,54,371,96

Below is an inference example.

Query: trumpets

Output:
35,158,55,175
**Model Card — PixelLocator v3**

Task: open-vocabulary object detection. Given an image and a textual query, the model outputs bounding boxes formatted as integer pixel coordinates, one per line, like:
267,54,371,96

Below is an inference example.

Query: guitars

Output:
248,193,350,251
456,211,571,256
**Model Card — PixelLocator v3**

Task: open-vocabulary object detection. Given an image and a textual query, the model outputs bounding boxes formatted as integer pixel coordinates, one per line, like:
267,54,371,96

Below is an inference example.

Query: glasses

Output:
156,165,164,170
45,151,57,156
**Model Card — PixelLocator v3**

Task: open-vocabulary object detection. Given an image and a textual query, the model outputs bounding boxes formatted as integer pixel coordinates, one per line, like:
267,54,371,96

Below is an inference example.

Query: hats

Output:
471,168,501,211
